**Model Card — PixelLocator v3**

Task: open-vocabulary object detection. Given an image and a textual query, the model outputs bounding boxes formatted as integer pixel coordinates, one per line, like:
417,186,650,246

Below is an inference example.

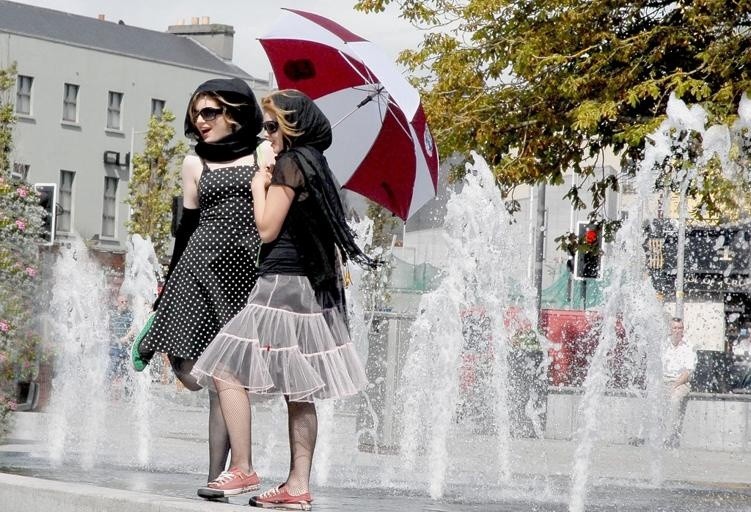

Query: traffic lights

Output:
574,221,605,280
32,184,56,247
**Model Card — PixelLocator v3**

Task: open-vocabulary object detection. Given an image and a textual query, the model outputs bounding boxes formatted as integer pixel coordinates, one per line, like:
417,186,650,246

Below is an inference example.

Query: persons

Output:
190,91,378,511
100,295,137,400
650,317,699,447
460,314,550,438
131,78,281,504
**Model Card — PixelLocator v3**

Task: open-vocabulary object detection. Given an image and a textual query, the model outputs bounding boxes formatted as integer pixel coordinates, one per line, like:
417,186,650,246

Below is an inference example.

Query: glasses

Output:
263,119,280,133
191,106,232,124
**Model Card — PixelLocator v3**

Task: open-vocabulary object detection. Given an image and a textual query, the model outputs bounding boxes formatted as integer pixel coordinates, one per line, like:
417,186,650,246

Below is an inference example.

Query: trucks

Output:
460,305,751,394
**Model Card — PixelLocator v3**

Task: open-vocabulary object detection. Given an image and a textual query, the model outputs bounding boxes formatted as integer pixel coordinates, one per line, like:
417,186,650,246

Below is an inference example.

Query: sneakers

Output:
628,438,680,449
248,482,313,510
197,467,261,498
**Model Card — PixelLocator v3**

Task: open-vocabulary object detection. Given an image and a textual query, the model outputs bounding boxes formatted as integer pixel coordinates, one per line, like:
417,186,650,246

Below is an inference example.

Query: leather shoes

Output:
132,312,157,371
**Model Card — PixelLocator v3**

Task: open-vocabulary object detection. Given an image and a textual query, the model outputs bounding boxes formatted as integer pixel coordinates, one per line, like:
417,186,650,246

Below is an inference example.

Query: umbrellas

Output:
257,6,440,224
